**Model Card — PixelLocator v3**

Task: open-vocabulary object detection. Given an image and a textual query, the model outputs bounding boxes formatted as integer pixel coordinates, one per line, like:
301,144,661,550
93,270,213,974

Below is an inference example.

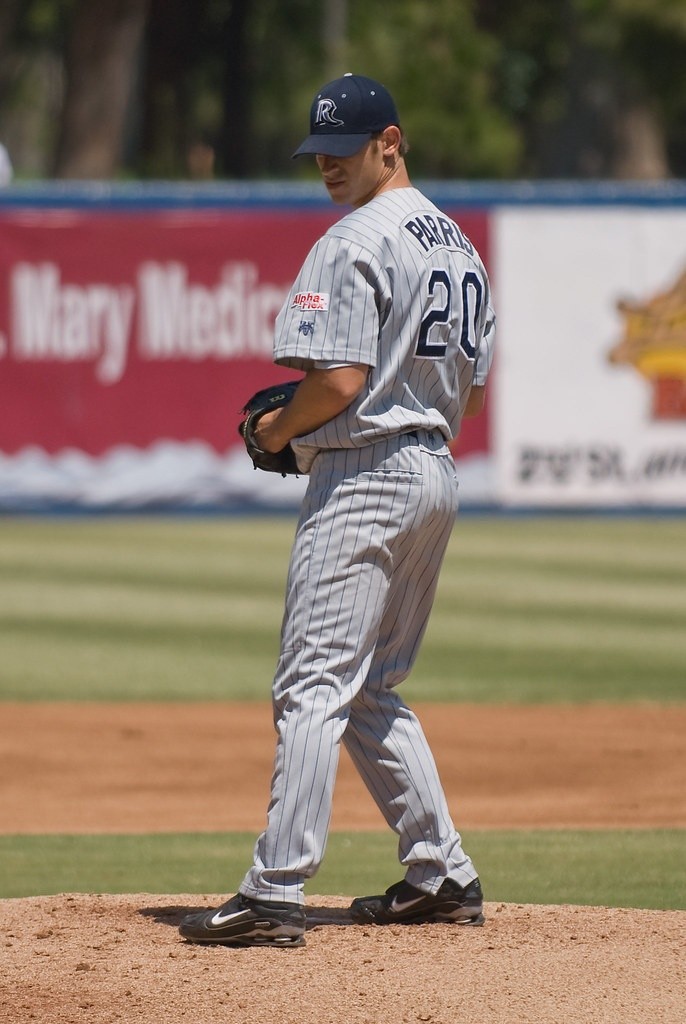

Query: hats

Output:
290,72,399,158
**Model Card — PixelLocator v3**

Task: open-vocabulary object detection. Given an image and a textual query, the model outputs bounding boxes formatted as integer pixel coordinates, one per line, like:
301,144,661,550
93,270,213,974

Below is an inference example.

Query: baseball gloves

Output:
237,378,315,480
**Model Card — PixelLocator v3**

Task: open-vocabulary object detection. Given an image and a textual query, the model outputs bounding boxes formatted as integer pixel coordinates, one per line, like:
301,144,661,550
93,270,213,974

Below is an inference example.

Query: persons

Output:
178,71,496,948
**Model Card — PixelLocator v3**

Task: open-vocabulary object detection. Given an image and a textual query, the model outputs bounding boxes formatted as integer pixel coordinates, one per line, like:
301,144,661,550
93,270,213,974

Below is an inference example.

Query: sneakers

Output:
350,877,485,927
179,893,306,947
